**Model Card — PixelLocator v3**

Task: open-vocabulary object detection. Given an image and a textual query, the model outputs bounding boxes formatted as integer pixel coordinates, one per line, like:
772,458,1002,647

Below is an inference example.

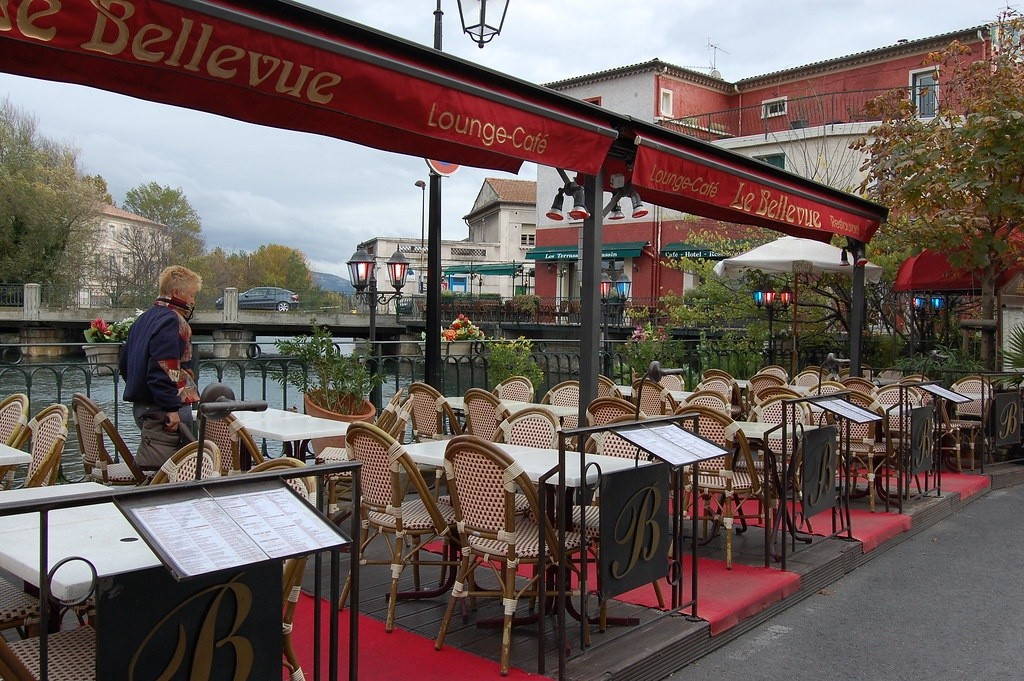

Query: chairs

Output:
0,623,96,680
0,359,995,680
847,105,867,123
396,299,663,324
0,576,40,639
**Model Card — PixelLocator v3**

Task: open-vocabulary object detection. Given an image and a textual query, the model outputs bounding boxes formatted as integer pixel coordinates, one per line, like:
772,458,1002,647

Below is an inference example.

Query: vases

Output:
80,345,126,377
418,340,484,364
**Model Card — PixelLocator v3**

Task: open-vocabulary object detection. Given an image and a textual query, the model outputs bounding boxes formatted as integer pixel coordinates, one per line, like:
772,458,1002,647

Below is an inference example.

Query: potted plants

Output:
788,91,810,127
269,316,387,459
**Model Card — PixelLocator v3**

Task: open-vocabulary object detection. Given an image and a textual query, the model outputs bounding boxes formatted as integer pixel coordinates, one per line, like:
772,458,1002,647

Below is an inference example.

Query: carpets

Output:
283,588,556,680
669,488,913,554
835,465,990,501
419,538,802,636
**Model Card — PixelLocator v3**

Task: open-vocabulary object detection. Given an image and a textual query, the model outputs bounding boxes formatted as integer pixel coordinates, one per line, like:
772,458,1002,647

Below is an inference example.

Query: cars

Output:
215,285,301,313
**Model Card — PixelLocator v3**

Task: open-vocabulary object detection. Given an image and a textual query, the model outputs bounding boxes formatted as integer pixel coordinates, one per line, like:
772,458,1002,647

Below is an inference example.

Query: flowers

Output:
83,308,147,344
421,313,486,340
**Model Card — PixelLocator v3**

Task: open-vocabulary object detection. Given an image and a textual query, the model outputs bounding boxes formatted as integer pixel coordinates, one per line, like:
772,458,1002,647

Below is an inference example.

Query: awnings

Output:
893,221,1024,361
525,241,647,259
661,238,778,259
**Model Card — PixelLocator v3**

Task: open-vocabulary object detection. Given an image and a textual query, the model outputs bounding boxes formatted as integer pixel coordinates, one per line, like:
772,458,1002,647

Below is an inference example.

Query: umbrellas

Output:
713,235,882,377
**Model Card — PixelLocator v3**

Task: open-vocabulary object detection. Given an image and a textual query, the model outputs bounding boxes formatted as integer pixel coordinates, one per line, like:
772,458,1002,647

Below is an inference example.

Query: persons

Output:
118,266,200,470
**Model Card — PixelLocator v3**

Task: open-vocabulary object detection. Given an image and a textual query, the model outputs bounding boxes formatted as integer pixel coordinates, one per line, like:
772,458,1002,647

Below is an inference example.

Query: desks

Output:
188,407,352,476
443,396,579,449
734,380,812,406
601,302,627,326
0,480,163,639
861,375,900,387
481,303,507,322
397,438,653,659
631,305,658,325
616,386,695,415
698,420,819,546
0,438,32,481
886,394,991,472
837,404,921,507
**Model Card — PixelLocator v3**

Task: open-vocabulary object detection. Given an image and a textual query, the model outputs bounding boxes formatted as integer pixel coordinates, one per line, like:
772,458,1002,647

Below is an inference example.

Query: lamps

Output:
567,181,589,219
753,283,765,309
546,182,569,220
608,191,625,219
839,243,852,265
779,284,791,309
623,186,648,218
915,293,925,310
850,243,868,266
441,263,631,300
385,244,411,291
913,292,919,308
347,241,376,288
762,280,777,309
930,293,944,312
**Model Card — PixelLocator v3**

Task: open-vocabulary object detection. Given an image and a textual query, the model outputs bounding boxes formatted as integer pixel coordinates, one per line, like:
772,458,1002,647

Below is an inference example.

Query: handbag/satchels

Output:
133,420,197,471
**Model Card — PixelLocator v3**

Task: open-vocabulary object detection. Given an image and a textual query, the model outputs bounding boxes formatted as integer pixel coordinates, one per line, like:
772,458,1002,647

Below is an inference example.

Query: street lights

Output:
601,270,632,382
914,291,944,357
753,281,793,366
345,242,411,404
415,180,426,294
